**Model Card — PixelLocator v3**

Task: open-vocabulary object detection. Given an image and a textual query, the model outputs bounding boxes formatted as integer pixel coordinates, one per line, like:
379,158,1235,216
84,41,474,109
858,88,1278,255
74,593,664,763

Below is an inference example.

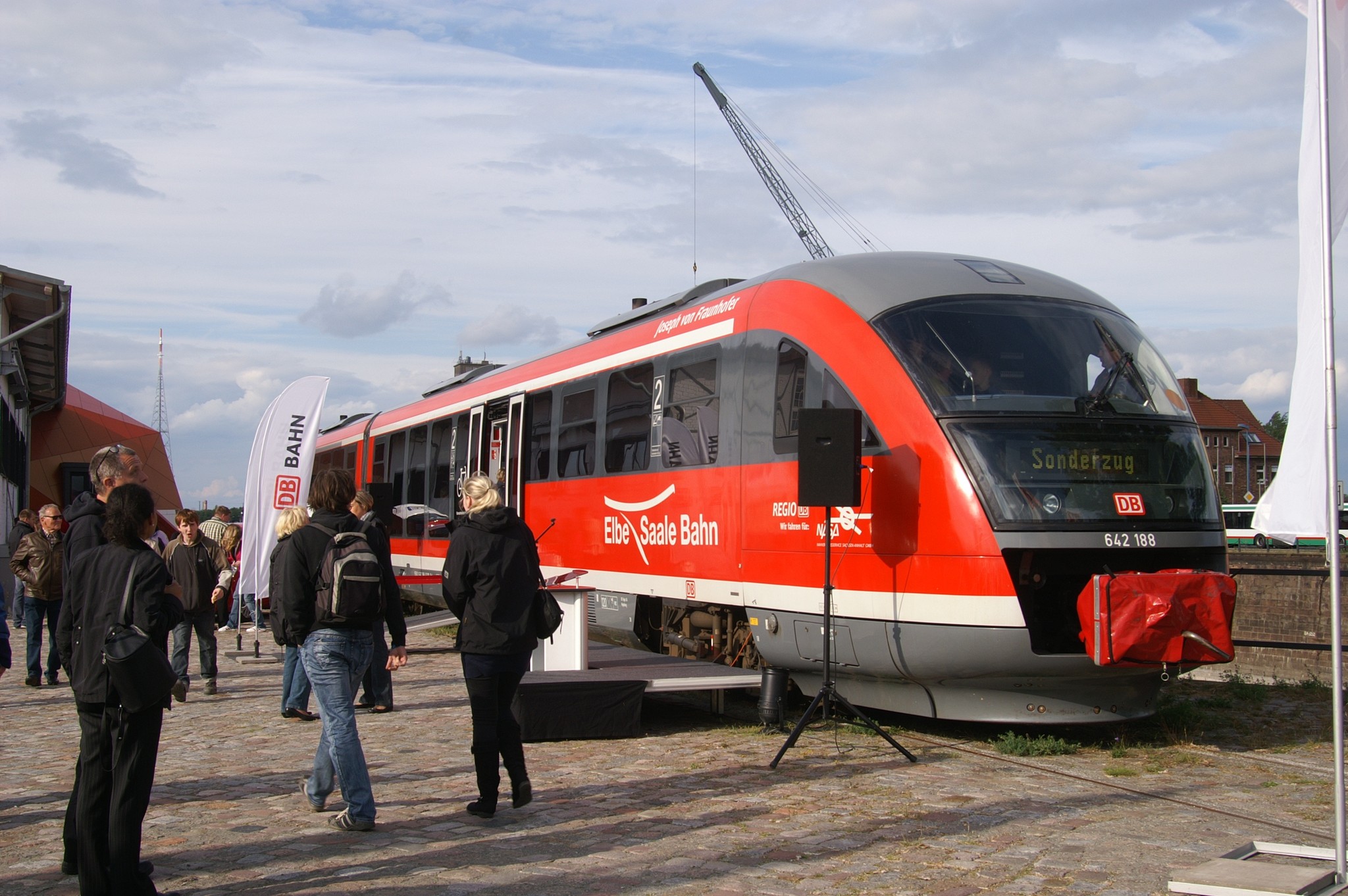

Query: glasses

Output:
43,515,64,520
96,444,124,483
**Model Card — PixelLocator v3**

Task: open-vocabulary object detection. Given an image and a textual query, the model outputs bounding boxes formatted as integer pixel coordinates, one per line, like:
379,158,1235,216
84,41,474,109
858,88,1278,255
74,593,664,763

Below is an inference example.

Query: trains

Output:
299,252,1240,731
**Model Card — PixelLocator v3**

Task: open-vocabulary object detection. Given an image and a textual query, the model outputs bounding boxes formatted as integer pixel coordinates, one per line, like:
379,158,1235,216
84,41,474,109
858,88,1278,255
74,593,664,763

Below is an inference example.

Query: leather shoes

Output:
354,703,371,708
281,707,321,721
367,706,393,713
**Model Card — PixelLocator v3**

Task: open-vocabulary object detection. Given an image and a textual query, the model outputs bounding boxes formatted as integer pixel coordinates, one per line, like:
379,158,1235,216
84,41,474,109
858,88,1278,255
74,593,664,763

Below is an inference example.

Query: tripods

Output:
768,507,918,769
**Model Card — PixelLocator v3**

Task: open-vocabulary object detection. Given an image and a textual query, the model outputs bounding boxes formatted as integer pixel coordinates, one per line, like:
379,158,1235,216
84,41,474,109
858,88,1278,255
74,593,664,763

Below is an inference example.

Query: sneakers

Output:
298,779,324,811
171,679,186,702
218,625,237,632
511,777,532,809
466,802,494,818
203,682,217,694
328,807,375,831
246,626,266,632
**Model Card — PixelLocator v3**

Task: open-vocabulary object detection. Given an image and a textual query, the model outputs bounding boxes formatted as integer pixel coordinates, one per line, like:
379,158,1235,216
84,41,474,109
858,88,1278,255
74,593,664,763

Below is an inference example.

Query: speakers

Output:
797,407,862,507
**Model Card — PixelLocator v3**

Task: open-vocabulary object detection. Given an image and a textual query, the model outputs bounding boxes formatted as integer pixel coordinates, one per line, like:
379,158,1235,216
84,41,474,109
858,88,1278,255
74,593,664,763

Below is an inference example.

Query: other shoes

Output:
139,859,154,875
47,679,59,685
25,676,41,687
61,861,79,875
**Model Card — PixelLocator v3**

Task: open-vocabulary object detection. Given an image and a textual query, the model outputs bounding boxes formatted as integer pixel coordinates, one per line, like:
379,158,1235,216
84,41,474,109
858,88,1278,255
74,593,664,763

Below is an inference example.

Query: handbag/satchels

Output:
524,525,564,639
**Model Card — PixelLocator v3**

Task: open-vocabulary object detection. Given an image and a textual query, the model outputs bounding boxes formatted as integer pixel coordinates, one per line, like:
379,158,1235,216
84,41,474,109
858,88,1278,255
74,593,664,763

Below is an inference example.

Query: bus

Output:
1222,503,1347,548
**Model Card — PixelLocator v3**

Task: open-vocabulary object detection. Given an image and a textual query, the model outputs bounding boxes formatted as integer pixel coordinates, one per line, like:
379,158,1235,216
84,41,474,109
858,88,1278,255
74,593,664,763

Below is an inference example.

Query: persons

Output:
1088,334,1149,404
927,347,1009,409
1,443,407,896
442,471,539,819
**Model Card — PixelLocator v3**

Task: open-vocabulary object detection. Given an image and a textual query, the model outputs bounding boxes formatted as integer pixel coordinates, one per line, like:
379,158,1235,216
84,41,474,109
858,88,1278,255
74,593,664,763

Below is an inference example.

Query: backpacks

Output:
305,519,382,631
100,547,178,769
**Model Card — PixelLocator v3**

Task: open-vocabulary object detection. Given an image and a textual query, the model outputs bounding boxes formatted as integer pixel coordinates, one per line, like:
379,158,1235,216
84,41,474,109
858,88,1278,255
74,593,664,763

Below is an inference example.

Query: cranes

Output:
688,58,833,263
